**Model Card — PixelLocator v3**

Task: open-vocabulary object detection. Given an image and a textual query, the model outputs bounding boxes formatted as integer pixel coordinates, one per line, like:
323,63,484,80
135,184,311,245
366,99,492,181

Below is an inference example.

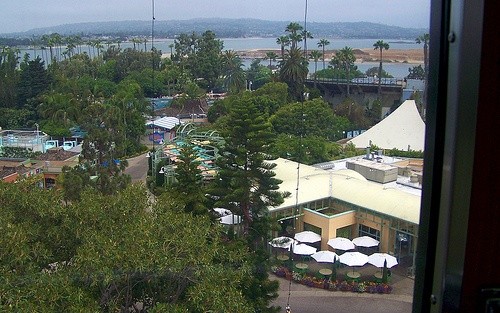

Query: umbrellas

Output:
310,250,338,271
338,252,368,275
367,252,398,275
218,214,243,225
290,243,317,267
268,237,295,258
328,238,356,252
351,235,380,251
294,230,322,246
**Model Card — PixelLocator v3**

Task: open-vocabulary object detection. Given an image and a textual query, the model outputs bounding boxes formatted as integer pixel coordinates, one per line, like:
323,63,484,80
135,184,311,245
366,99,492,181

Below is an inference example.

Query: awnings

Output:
145,116,182,136
